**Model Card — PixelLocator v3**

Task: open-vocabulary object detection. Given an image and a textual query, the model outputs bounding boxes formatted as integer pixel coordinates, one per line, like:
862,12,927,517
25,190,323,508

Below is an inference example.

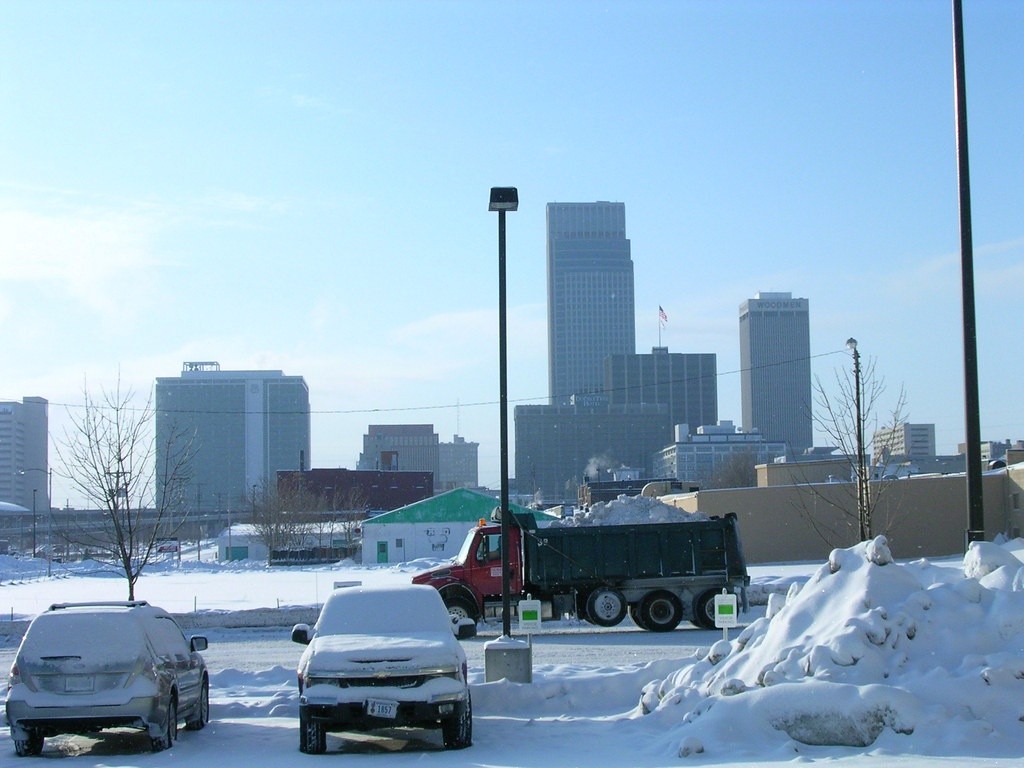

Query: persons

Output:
479,536,501,560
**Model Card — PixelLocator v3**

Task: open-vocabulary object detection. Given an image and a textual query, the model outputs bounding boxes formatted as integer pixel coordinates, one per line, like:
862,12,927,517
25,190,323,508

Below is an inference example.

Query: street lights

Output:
250,484,258,525
486,183,524,643
32,489,38,559
17,465,52,576
846,337,867,544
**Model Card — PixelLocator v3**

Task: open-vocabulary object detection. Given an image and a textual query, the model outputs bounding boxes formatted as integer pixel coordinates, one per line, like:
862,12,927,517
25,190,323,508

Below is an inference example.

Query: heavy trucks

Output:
410,503,752,638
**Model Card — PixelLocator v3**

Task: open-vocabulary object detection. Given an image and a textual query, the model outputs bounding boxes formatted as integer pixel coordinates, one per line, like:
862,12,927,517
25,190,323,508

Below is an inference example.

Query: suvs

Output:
5,600,213,753
287,586,478,753
